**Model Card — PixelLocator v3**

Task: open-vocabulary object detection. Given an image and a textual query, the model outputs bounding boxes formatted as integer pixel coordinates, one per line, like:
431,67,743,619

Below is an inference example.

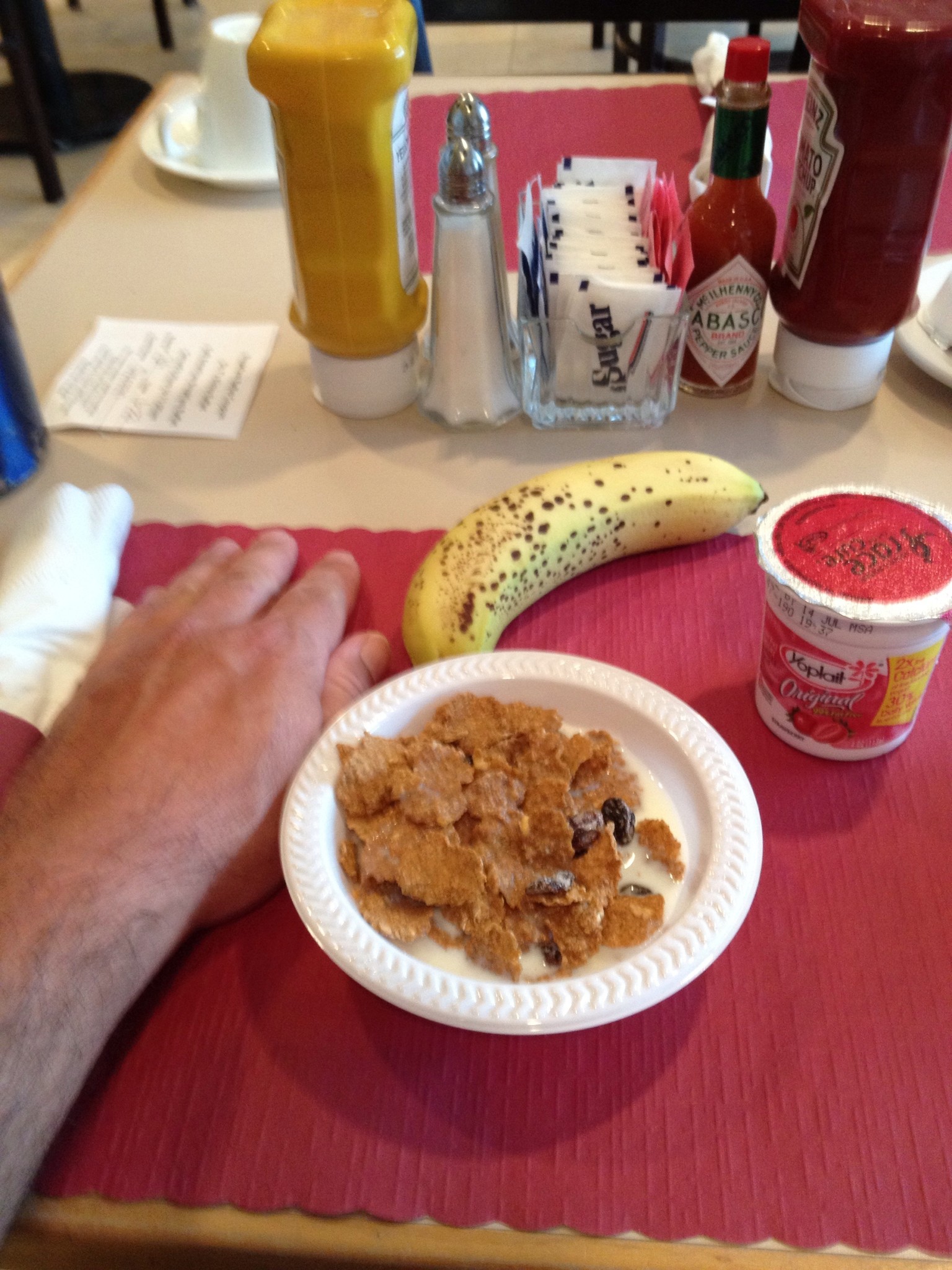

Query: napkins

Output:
0,483,137,741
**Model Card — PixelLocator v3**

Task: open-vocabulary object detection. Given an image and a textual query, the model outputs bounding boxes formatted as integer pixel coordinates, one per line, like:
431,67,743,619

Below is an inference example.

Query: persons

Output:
0,528,390,1242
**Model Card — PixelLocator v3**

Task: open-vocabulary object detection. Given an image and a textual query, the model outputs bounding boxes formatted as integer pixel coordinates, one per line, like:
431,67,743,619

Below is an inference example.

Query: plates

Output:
897,255,951,384
278,649,764,1037
138,90,286,193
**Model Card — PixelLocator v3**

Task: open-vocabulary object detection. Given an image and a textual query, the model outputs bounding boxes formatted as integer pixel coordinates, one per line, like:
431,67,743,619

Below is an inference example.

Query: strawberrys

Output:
787,707,854,743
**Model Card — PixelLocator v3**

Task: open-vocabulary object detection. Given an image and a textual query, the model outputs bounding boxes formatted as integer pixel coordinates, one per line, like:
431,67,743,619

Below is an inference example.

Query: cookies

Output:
334,692,686,982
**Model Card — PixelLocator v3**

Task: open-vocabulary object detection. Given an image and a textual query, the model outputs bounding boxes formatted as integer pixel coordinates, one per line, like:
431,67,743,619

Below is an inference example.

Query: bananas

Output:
403,450,770,665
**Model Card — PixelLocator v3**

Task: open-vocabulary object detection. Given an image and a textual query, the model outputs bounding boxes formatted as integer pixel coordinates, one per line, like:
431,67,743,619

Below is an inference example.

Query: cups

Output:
194,14,276,167
1,278,49,496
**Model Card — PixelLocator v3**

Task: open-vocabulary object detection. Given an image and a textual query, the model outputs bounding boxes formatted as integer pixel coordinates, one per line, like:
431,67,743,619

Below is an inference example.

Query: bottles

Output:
245,0,431,415
421,93,526,428
678,36,780,396
761,1,951,412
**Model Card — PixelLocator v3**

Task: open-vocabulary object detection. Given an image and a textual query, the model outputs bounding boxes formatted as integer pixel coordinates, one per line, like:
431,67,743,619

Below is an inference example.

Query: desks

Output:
0,25,951,1270
